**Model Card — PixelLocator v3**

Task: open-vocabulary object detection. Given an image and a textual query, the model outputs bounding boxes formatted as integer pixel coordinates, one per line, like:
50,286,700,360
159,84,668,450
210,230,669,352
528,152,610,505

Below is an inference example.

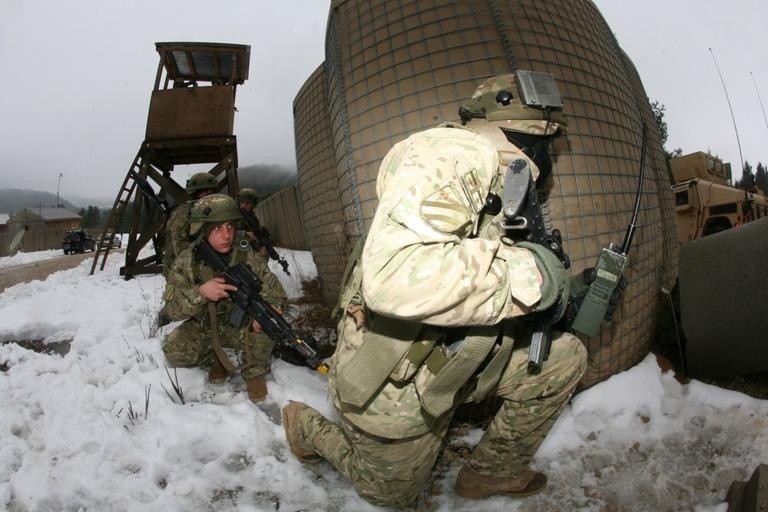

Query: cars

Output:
97,234,121,248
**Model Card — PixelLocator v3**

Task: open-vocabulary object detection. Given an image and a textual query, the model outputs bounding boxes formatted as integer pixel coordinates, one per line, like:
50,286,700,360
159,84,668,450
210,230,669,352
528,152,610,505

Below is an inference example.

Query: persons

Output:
234,187,266,242
284,69,588,507
162,192,287,403
162,172,219,281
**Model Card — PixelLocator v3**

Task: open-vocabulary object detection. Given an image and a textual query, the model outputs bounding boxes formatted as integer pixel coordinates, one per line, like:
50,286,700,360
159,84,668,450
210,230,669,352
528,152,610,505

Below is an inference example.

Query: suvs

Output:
62,229,95,254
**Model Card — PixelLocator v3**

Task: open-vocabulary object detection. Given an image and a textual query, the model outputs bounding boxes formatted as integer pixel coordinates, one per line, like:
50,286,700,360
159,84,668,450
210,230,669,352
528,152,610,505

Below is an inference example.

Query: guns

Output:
197,241,322,370
238,207,294,276
502,158,572,372
131,168,172,214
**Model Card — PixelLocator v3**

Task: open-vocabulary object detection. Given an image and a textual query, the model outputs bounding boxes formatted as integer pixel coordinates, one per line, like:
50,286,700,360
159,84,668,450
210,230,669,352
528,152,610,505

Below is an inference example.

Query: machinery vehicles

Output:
668,152,768,243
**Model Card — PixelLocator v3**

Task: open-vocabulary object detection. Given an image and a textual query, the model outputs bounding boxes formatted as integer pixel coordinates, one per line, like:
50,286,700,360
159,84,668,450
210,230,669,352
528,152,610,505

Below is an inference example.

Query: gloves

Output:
512,241,571,326
563,268,627,322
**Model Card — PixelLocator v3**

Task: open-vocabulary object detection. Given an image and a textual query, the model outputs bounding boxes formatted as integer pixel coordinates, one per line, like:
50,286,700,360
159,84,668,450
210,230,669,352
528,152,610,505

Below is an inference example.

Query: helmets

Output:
458,74,569,135
186,173,218,194
237,187,258,204
189,193,242,223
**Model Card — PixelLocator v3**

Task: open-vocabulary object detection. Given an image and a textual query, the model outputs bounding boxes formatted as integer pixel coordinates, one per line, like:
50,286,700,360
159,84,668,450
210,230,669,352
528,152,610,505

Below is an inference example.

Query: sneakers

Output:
455,469,547,499
208,364,226,384
247,377,267,401
281,401,323,464
